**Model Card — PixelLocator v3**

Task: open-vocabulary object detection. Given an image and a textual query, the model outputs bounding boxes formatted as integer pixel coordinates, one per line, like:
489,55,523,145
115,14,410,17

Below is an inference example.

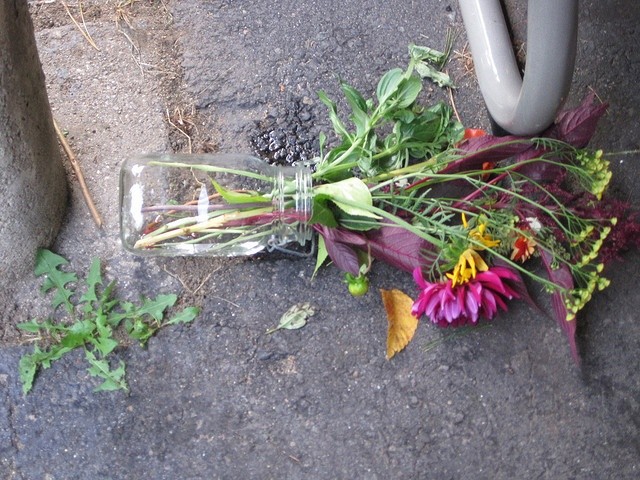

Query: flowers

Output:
133,42,635,365
